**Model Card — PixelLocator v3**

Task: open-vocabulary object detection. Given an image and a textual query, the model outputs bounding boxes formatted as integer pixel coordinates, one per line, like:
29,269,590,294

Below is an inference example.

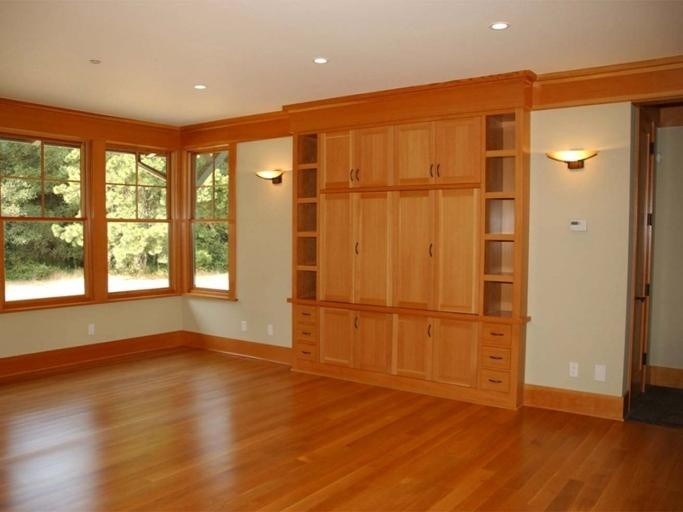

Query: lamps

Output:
542,151,600,170
255,169,284,184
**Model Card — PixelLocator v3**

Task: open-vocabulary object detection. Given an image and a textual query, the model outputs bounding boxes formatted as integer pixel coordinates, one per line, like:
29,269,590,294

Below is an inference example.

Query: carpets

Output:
627,385,682,428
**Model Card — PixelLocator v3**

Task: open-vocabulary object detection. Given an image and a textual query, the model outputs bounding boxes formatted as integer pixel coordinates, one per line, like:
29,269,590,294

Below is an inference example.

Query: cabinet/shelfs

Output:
292,131,320,302
320,127,395,191
478,322,522,409
320,189,392,304
393,311,478,389
292,302,319,364
392,117,482,186
392,187,478,317
484,109,522,319
319,303,392,374
